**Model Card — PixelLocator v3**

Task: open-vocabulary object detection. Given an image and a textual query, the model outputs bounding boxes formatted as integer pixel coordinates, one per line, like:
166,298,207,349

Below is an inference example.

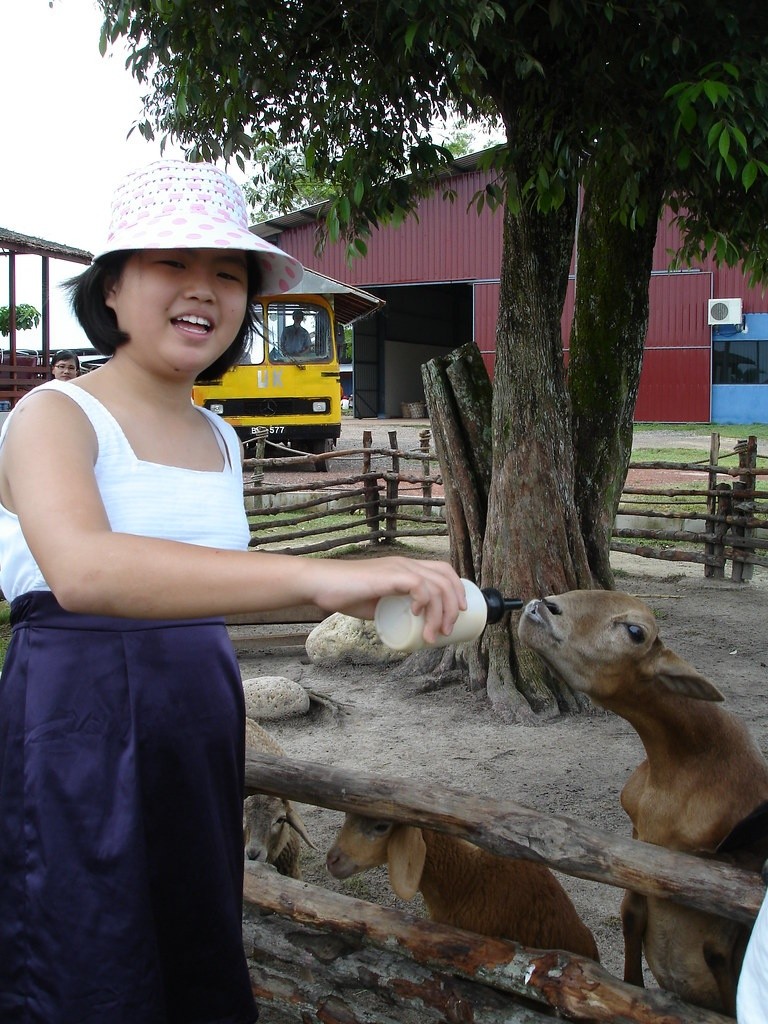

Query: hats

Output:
90,161,303,297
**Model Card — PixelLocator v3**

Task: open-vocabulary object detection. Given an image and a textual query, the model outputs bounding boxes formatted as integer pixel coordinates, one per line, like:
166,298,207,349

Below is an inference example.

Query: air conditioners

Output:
707,298,743,326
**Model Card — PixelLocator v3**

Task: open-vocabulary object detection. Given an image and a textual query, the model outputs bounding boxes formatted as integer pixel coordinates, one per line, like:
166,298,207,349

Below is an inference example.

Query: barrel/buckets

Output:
401,401,424,419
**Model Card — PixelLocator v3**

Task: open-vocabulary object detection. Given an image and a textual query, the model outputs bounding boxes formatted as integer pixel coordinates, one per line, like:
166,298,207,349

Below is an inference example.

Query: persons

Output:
0,160,469,1024
50,349,81,381
281,309,312,361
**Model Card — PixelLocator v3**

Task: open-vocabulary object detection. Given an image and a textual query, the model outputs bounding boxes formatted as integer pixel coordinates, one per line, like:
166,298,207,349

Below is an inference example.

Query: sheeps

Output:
243,795,324,881
518,589,768,1016
328,812,598,964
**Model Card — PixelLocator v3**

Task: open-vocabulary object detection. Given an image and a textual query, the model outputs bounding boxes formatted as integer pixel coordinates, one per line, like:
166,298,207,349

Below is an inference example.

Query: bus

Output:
191,293,343,476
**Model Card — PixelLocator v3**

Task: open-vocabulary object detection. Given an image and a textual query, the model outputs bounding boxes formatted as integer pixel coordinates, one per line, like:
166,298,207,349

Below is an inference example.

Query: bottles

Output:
375,575,525,650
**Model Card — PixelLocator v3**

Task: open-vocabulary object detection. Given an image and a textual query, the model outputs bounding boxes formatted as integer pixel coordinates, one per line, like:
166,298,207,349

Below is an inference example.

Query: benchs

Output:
0,355,37,410
